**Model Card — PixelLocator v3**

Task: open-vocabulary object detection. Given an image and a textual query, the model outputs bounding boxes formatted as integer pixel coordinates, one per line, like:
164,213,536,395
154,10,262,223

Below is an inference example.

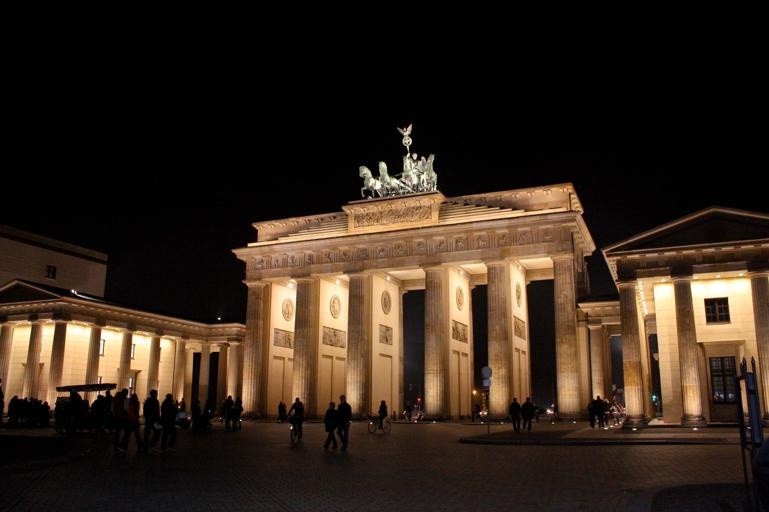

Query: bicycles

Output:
367,411,393,435
288,415,299,445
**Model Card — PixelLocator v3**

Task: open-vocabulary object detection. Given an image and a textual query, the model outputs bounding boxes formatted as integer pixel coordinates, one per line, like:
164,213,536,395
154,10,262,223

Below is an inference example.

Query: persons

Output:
588,393,624,430
0,385,243,458
378,400,388,429
407,151,424,167
336,395,352,451
405,399,412,421
471,406,481,422
322,402,339,451
278,402,287,423
287,397,303,442
506,397,560,434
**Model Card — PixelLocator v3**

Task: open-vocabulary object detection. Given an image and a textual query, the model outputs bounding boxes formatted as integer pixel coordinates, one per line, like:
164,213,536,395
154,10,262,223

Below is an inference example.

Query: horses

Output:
402,154,421,192
378,161,399,195
424,153,438,190
358,165,381,198
